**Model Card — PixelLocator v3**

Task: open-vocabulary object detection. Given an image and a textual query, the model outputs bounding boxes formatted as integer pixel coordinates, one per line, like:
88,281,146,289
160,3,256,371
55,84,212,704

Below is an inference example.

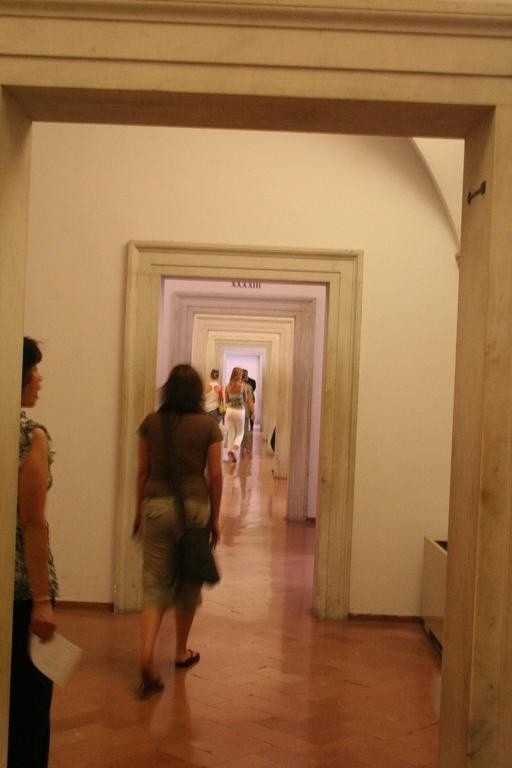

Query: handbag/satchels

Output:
218,403,227,413
173,523,219,584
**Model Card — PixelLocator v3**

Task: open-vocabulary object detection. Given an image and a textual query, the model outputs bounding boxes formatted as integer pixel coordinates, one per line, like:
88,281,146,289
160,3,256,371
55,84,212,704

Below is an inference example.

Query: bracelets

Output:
32,594,51,602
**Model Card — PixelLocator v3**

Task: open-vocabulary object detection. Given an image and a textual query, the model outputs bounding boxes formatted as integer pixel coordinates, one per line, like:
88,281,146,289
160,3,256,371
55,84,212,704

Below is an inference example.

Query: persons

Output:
5,337,59,768
129,365,223,698
204,462,251,546
202,368,255,462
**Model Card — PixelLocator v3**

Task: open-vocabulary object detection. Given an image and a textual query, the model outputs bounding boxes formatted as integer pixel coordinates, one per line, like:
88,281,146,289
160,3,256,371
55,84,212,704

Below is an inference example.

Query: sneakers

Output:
228,450,237,463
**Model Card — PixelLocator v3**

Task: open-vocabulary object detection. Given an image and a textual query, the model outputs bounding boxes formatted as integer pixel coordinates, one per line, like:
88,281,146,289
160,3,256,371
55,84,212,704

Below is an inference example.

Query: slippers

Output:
174,649,200,669
140,664,163,691
